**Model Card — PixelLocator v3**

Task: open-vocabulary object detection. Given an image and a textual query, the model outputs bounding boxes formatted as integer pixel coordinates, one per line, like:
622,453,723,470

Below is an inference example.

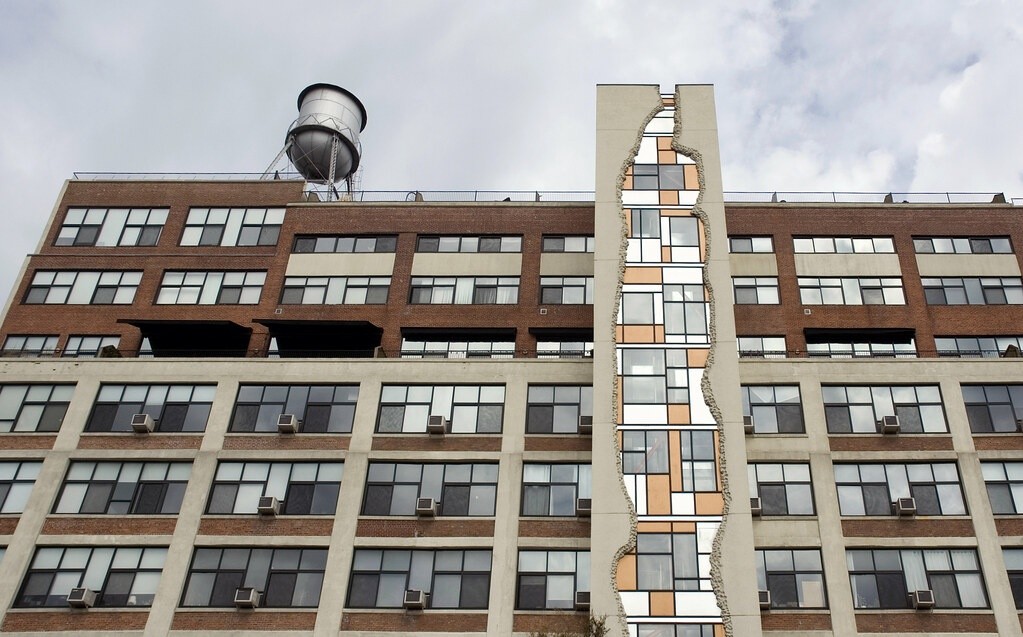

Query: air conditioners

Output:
579,415,592,432
758,590,771,608
576,591,591,610
276,414,300,433
577,498,591,516
415,497,436,516
67,588,98,608
131,414,156,434
744,415,754,433
881,416,901,436
750,497,762,518
233,588,261,609
896,497,917,521
914,589,936,609
404,590,427,610
257,496,281,515
426,415,448,432
